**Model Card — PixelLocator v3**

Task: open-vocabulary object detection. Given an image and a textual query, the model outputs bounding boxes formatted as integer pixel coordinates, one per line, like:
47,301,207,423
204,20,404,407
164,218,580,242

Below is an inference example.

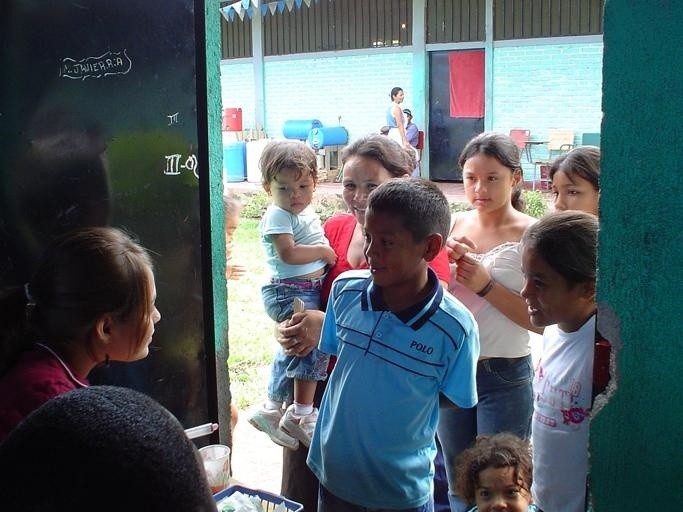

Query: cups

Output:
8,133,114,257
235,127,266,143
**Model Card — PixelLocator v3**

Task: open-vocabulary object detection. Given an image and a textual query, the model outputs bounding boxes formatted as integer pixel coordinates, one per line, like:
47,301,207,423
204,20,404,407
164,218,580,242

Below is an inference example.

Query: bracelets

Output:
476,278,495,298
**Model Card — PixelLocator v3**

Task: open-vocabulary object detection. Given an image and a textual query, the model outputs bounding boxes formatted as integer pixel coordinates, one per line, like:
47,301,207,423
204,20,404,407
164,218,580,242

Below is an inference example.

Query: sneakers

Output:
278,404,319,448
247,403,300,451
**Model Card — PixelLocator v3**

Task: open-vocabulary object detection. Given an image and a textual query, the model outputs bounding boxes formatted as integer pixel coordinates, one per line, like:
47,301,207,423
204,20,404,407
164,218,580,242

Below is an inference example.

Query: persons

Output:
220,192,245,431
0,386,219,512
442,132,545,512
402,109,419,148
386,87,408,148
304,176,481,512
547,144,599,219
0,228,161,441
280,134,451,512
518,209,598,512
247,140,337,450
450,432,543,512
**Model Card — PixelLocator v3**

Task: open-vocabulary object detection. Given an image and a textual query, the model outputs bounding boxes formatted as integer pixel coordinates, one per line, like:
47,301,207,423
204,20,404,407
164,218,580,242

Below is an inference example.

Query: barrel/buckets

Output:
282,120,323,140
308,127,348,149
223,107,242,131
222,140,247,182
246,139,270,182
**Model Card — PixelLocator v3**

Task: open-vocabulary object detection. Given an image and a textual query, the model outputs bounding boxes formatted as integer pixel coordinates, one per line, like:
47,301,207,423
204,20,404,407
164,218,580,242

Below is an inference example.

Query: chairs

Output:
412,130,424,177
505,129,529,193
581,133,603,150
534,130,575,191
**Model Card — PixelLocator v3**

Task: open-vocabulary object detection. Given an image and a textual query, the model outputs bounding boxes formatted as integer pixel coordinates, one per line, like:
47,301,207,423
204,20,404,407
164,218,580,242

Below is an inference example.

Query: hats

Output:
403,109,412,118
